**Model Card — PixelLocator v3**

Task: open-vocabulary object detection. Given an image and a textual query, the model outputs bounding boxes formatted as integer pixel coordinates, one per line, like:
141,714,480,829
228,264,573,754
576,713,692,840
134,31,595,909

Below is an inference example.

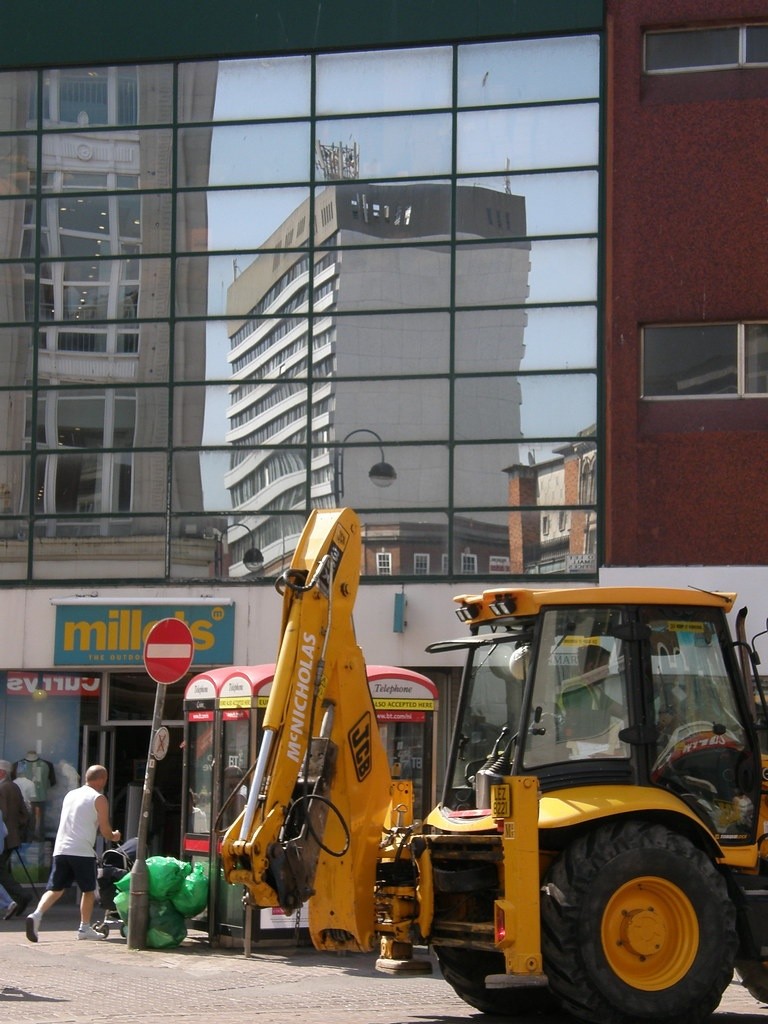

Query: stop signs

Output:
143,618,194,685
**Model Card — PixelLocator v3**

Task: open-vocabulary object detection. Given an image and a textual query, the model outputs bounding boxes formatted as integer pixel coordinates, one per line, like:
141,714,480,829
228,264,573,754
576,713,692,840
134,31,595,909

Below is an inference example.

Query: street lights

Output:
332,425,399,508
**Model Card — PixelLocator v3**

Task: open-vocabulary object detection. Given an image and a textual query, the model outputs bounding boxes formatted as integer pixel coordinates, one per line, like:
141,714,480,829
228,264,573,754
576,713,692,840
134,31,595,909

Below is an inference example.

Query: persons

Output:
25,765,121,942
186,790,207,834
0,760,32,920
222,766,248,825
13,777,37,843
557,644,672,745
11,751,57,841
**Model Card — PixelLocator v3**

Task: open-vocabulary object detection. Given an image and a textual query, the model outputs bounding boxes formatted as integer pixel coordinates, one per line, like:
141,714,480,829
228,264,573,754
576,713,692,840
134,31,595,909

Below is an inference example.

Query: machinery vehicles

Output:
211,506,767,1024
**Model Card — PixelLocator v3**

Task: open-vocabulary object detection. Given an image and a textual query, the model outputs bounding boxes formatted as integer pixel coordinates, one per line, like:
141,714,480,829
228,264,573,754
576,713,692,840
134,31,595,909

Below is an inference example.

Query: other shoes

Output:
16,893,34,916
2,903,17,920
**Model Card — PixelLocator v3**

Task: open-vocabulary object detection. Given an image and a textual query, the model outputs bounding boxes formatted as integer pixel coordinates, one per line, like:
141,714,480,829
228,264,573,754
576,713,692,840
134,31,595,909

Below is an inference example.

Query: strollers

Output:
92,836,152,941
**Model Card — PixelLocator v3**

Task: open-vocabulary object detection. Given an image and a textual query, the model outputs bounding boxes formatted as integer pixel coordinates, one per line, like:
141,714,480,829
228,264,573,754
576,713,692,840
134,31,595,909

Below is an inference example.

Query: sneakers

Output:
26,914,40,942
77,927,105,941
